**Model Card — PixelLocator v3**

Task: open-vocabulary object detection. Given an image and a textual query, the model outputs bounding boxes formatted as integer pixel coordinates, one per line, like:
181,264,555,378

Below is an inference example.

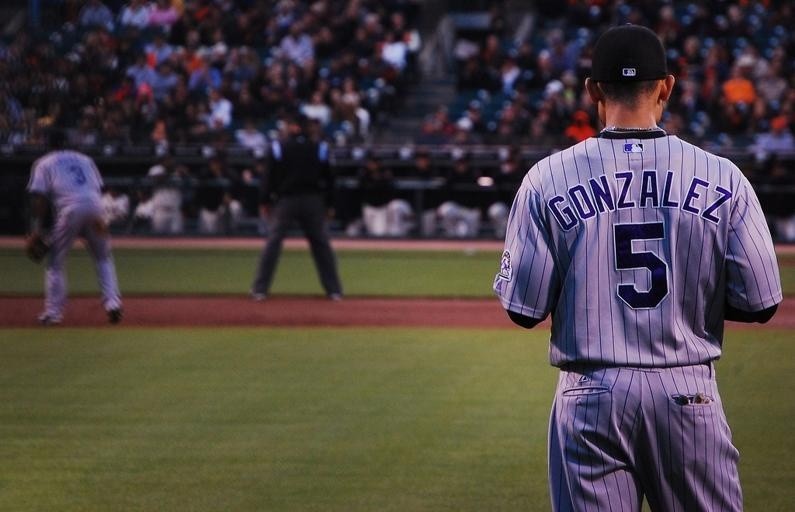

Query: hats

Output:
592,23,668,84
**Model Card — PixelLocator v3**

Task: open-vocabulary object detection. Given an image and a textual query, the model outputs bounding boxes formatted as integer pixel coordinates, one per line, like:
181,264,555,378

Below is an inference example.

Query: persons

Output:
2,0,794,248
491,23,785,510
242,113,348,303
20,131,126,322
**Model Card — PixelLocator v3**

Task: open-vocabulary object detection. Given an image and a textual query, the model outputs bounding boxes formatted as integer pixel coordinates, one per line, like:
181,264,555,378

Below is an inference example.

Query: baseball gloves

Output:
24,230,51,264
